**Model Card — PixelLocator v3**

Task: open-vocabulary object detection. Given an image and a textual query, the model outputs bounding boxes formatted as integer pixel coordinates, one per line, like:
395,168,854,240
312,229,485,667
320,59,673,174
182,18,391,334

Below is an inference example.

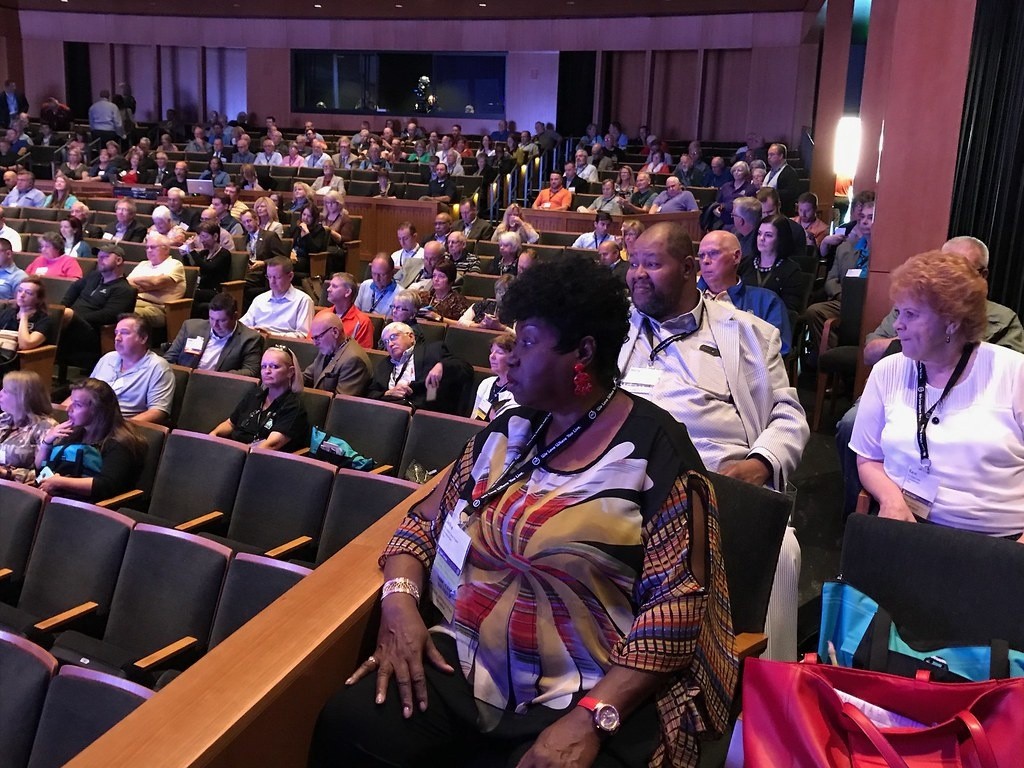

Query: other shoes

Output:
824,371,837,395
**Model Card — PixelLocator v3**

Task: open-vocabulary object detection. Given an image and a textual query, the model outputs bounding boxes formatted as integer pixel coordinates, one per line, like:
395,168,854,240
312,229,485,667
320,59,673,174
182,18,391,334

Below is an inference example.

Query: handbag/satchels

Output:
820,583,1023,683
310,427,375,472
741,653,1024,768
41,439,102,504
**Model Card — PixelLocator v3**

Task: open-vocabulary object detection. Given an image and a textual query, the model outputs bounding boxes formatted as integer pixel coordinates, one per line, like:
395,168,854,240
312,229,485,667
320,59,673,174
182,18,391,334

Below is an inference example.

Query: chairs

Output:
0,117,1024,768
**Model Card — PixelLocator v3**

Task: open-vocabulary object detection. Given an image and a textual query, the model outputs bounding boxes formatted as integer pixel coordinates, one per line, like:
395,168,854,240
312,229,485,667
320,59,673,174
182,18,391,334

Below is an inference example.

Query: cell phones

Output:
40,466,53,478
427,384,437,401
619,192,625,199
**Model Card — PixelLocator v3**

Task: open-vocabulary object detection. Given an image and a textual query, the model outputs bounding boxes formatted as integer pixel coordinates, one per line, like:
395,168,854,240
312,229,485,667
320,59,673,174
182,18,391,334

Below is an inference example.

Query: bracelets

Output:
382,576,420,603
41,439,52,445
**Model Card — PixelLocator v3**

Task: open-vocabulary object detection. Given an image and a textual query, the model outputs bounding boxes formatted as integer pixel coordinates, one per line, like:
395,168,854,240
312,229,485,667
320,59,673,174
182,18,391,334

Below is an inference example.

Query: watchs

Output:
577,695,623,736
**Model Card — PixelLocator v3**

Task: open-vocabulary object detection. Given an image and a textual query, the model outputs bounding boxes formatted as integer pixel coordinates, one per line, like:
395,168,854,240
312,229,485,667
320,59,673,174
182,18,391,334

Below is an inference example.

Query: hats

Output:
91,244,125,258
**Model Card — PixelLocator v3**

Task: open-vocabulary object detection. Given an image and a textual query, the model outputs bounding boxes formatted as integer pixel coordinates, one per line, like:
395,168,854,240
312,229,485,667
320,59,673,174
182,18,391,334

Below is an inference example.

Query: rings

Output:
368,656,376,664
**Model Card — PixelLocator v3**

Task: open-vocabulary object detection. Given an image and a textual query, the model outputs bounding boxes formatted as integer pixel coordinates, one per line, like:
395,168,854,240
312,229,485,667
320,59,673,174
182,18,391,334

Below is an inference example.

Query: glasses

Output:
311,327,331,340
730,212,740,218
390,304,410,311
384,333,397,345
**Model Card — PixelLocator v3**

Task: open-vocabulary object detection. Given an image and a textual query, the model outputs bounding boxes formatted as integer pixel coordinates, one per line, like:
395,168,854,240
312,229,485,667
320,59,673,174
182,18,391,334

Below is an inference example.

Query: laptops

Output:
186,178,215,196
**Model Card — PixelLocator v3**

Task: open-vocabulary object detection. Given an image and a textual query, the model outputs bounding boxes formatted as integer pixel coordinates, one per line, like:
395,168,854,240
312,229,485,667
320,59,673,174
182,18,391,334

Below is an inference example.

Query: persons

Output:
838,234,1024,543
346,249,737,768
0,72,876,499
